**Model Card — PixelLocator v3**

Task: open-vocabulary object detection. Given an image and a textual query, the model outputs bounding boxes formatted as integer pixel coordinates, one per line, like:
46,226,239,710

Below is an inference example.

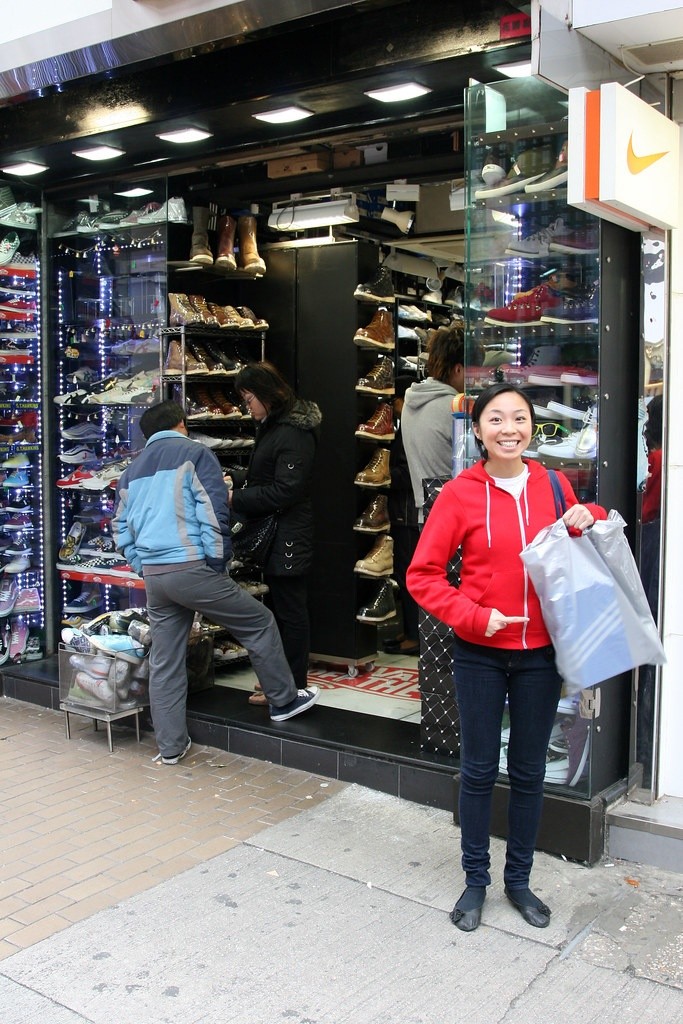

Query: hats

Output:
390,374,420,398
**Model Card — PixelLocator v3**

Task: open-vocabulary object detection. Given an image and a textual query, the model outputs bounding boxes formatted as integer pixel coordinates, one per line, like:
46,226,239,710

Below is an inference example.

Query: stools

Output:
60,703,146,754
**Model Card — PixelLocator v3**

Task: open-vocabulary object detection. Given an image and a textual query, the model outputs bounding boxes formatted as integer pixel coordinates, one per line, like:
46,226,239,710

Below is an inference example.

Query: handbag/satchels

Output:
242,515,278,560
519,507,668,696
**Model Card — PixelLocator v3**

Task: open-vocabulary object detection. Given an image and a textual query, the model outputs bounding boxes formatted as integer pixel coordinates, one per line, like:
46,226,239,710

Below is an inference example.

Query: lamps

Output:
362,80,435,104
0,161,49,177
72,145,127,161
252,105,316,123
266,187,360,230
381,177,420,234
154,126,214,143
492,60,531,78
447,179,485,212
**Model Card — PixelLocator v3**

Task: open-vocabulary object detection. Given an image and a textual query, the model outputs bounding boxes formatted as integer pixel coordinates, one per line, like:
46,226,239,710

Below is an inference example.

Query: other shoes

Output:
248,691,268,704
254,683,263,690
449,885,486,932
504,885,551,927
384,640,420,656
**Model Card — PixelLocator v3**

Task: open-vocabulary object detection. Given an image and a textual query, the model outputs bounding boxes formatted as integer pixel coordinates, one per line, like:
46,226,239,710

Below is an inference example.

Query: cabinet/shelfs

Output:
0,193,267,675
357,120,601,768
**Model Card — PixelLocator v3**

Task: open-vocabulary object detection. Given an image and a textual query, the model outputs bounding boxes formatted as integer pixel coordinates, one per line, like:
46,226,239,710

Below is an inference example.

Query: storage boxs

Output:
58,632,217,713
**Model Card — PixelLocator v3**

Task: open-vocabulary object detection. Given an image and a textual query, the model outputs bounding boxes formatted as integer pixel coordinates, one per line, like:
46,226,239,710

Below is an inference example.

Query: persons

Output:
398,328,485,530
637,395,662,523
112,398,319,765
223,360,324,705
406,385,608,933
388,369,421,659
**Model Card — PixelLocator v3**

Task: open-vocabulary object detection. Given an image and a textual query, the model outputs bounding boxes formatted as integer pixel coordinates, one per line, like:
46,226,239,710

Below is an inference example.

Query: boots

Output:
161,205,269,488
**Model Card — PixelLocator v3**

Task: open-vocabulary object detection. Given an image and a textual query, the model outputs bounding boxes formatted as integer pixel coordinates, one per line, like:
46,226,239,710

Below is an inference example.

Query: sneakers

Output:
354,449,391,487
354,357,396,395
355,579,397,622
498,693,583,782
399,141,600,457
162,736,192,764
187,558,270,661
353,266,395,303
354,402,395,440
269,684,320,721
353,494,390,532
353,310,396,350
0,173,191,716
353,535,393,576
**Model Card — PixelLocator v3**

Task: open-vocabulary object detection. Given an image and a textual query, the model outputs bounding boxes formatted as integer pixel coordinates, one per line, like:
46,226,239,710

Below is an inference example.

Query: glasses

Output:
242,394,255,407
532,422,571,438
389,397,399,405
